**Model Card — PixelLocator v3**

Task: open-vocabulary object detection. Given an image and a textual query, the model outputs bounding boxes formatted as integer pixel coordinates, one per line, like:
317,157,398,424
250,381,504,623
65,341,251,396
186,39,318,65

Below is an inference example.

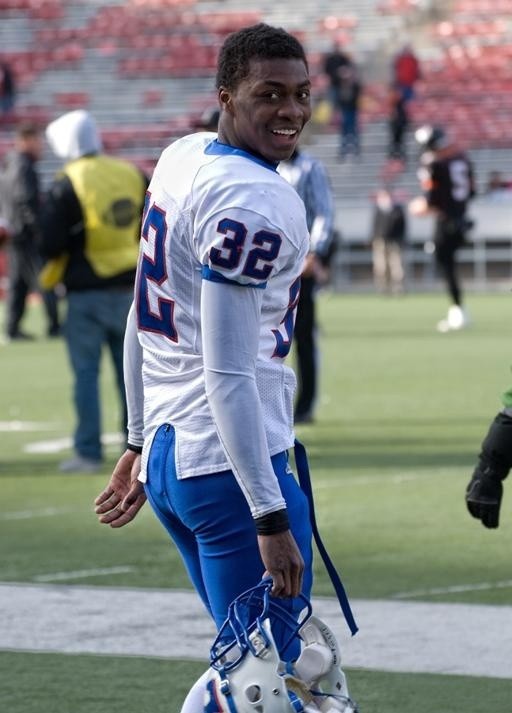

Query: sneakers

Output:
57,452,99,472
438,306,466,332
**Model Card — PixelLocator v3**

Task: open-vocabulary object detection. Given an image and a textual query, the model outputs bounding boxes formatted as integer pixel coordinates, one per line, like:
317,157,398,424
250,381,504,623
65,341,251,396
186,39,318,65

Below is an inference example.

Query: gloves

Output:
468,414,510,528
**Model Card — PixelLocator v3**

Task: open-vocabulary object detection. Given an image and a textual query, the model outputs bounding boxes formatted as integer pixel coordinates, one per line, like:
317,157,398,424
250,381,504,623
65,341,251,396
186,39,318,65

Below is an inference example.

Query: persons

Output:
464,384,511,528
314,38,422,175
95,20,359,662
0,121,65,340
406,124,480,337
273,145,337,425
30,106,151,473
368,186,405,303
188,103,220,130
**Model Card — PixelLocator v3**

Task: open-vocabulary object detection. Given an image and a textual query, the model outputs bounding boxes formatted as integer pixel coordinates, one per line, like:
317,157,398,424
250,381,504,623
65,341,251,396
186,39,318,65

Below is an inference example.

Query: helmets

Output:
46,107,101,161
415,123,446,151
180,575,354,713
